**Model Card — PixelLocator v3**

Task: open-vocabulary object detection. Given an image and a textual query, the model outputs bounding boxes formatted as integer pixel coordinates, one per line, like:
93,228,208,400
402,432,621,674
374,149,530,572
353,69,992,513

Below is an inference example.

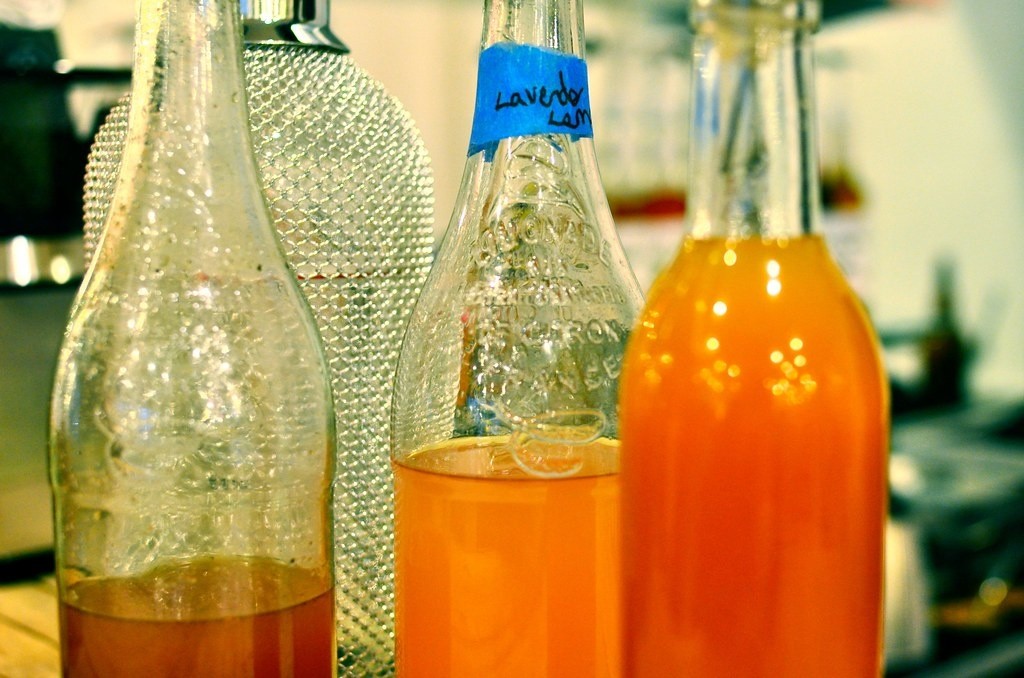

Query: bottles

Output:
391,0,684,678
79,0,429,678
49,0,343,678
618,0,889,678
